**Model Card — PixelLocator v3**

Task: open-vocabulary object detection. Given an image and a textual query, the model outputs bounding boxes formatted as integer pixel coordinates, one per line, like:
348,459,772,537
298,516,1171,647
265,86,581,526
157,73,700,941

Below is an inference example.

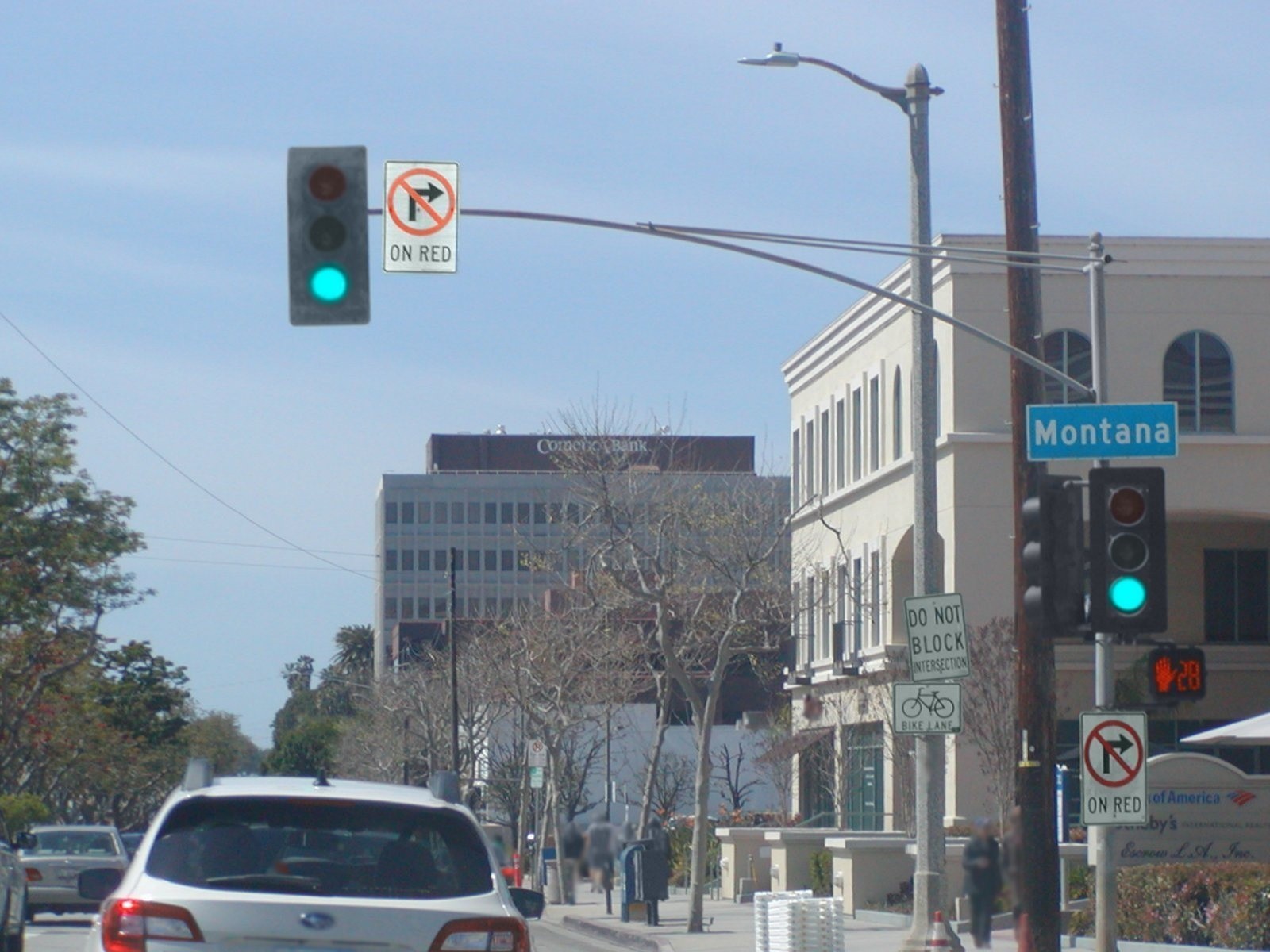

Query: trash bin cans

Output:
544,859,574,904
620,844,640,924
635,839,669,925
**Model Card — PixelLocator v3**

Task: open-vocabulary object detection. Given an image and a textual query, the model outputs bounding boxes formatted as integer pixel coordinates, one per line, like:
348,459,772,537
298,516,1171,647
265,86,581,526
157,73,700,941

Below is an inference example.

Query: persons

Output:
493,818,676,921
961,818,1004,950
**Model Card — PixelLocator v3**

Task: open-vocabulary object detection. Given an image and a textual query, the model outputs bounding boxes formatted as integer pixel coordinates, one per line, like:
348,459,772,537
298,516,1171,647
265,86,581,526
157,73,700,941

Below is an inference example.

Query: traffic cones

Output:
930,910,954,952
1018,914,1037,951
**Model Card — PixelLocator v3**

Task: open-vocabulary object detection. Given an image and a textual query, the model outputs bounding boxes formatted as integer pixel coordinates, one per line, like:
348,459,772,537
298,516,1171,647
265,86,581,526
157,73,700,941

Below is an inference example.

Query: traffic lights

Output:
283,148,370,327
1084,465,1166,637
1148,644,1207,696
1019,471,1083,635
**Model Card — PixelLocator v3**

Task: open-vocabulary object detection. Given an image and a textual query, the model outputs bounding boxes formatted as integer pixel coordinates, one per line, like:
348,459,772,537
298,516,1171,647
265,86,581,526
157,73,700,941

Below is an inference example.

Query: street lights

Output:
740,43,964,952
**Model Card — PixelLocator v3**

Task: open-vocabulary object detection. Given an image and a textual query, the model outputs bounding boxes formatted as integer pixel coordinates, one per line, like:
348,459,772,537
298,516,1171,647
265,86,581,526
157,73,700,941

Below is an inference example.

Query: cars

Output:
0,824,145,952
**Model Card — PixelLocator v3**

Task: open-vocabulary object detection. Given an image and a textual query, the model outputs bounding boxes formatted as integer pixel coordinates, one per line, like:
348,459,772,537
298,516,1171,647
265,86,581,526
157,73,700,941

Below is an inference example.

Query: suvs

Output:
92,759,545,952
